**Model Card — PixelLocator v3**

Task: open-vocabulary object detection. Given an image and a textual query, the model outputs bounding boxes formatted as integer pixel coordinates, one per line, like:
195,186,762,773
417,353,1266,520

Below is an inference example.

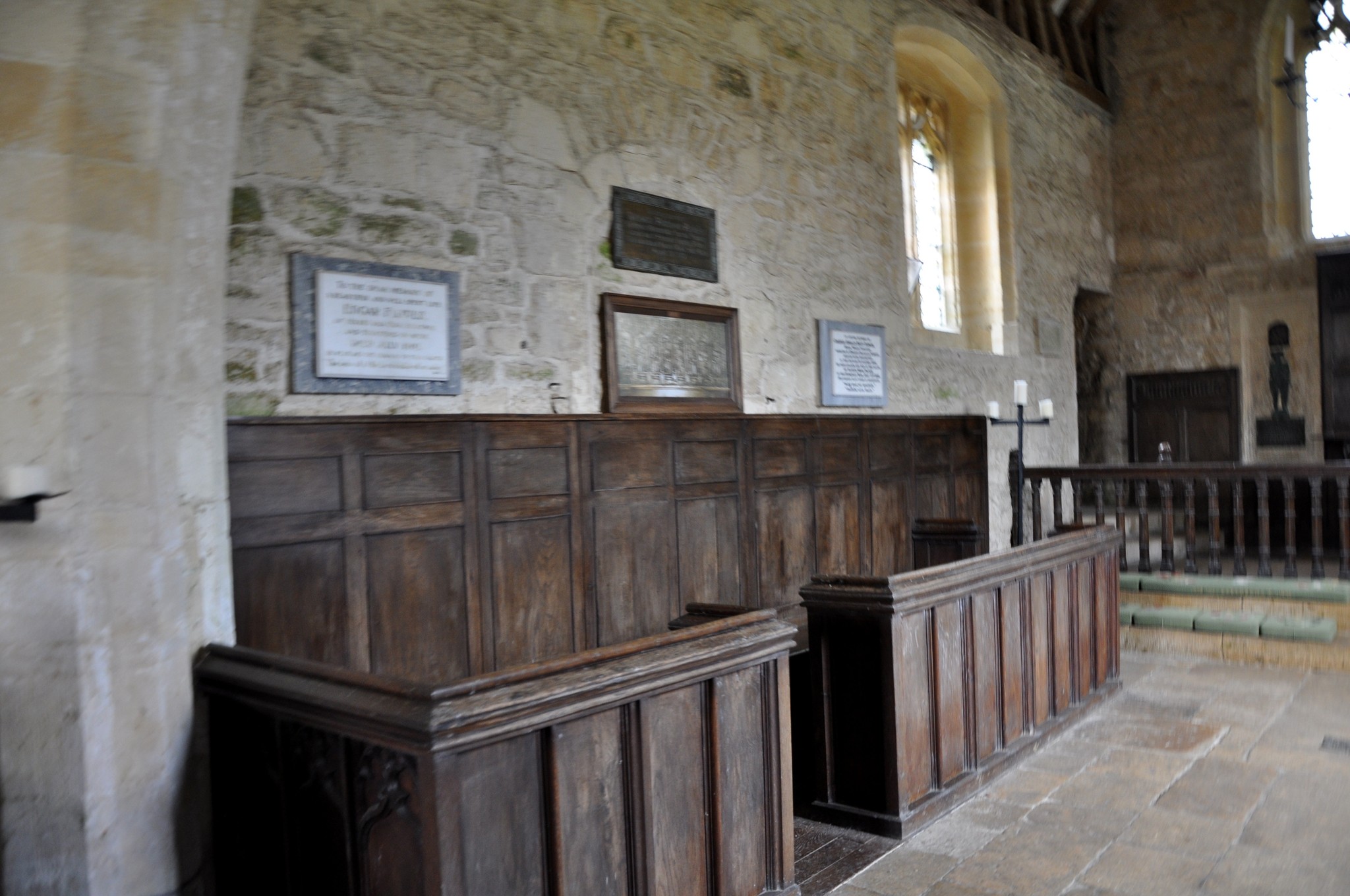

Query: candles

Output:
986,401,999,419
3,466,47,498
1038,398,1053,419
1013,380,1028,406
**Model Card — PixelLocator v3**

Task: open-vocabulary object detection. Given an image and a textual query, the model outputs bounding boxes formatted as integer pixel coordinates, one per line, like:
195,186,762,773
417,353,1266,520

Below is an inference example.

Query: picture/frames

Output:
600,292,743,413
